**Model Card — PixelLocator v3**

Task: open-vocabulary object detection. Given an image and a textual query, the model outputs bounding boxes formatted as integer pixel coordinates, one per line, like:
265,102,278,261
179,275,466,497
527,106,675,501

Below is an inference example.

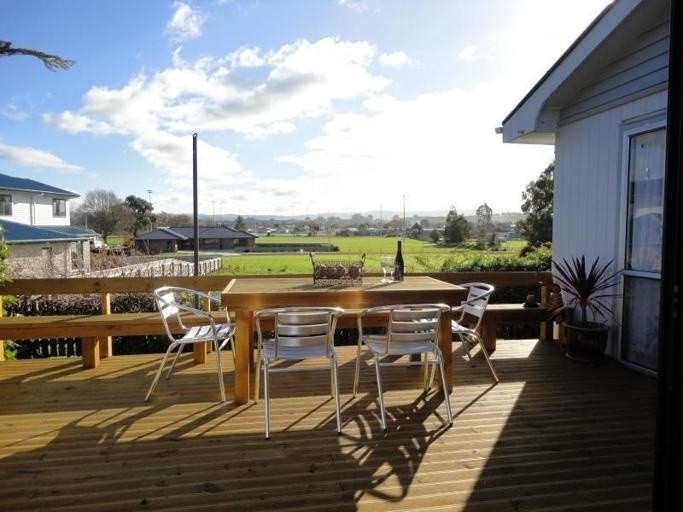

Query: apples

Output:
316,265,360,279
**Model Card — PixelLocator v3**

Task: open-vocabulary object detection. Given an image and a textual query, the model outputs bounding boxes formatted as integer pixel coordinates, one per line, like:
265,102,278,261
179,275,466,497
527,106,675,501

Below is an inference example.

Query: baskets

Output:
309,252,367,285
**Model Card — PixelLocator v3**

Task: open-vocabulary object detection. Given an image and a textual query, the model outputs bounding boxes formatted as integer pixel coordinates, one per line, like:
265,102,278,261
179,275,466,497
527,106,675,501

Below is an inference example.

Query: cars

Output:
92,246,130,256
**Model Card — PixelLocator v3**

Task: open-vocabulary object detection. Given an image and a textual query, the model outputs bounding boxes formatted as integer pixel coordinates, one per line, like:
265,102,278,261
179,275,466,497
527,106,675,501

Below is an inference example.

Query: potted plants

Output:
546,253,625,359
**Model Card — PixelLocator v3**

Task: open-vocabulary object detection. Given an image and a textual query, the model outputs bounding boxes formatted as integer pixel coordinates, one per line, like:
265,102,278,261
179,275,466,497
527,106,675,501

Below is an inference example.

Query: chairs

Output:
352,303,454,433
250,306,347,439
426,282,498,392
145,286,240,404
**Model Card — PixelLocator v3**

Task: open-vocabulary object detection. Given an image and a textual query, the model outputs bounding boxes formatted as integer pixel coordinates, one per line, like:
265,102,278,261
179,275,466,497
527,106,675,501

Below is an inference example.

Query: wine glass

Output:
380,256,396,284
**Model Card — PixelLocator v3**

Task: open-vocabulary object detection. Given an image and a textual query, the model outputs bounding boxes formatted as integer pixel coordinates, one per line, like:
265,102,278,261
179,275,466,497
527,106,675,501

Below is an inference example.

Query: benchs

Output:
1,269,555,385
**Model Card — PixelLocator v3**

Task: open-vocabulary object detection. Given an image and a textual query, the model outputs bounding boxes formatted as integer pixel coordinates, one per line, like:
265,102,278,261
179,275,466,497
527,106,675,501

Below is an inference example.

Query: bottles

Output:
392,240,404,281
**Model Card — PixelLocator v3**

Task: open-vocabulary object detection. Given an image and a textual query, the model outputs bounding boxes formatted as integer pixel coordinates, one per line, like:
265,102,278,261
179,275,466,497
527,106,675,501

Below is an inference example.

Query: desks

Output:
220,275,469,405
